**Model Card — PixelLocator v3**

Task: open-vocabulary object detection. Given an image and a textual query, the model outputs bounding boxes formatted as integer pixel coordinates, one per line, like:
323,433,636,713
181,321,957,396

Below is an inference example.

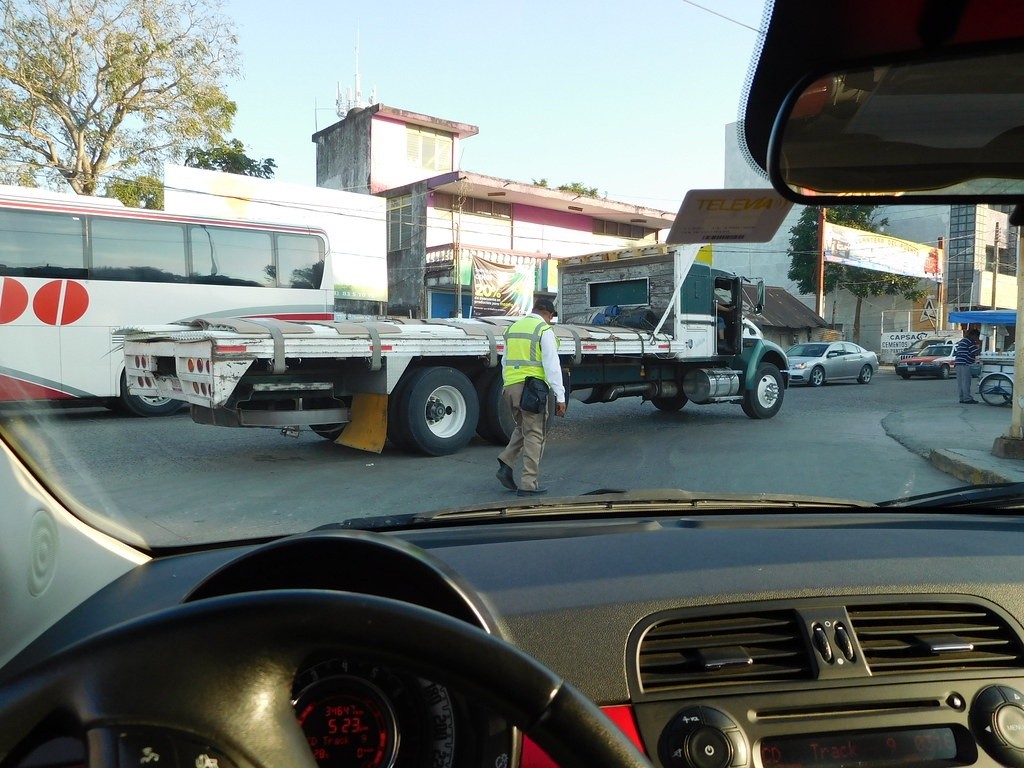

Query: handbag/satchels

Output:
519,377,549,413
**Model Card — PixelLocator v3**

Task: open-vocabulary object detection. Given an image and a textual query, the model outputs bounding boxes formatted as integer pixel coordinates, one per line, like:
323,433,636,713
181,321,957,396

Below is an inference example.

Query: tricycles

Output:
948,308,1018,408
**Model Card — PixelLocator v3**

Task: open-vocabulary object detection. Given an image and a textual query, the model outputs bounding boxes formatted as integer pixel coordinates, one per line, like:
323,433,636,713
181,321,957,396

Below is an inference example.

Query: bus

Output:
0,184,337,418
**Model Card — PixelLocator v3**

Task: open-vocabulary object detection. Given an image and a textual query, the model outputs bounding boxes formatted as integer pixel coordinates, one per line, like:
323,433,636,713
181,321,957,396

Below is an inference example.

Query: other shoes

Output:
718,339,733,352
960,399,979,404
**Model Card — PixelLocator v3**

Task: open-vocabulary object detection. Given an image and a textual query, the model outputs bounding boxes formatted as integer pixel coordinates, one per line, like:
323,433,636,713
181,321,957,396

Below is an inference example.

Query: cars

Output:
781,340,879,387
895,344,959,381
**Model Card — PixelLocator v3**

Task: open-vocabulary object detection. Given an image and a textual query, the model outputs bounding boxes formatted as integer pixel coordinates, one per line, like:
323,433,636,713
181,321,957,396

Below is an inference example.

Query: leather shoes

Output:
496,465,518,491
517,486,548,497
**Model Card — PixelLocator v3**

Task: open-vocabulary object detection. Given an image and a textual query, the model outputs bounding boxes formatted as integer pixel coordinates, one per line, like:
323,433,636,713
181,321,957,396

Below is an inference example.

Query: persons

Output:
496,298,567,496
954,329,981,404
714,302,737,344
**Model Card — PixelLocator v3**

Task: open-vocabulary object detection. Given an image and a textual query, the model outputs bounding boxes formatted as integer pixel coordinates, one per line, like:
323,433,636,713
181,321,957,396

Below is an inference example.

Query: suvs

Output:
892,336,952,375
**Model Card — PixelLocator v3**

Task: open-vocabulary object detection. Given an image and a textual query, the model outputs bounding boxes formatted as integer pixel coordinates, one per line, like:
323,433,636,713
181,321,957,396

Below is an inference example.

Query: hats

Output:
535,299,558,317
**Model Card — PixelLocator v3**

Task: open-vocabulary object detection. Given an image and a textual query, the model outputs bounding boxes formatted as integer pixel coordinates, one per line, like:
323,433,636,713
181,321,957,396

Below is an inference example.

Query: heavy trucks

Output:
120,238,792,457
